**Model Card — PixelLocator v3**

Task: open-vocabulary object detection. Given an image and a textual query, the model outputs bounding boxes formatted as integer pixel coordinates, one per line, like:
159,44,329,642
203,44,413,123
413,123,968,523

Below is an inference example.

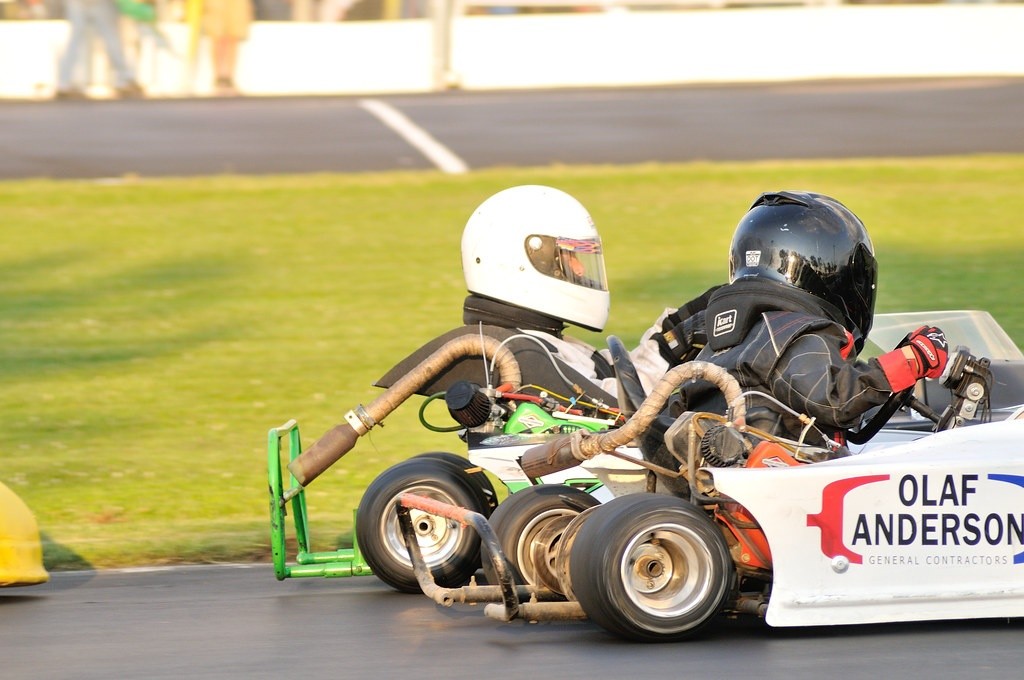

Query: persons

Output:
661,189,950,456
56,0,148,101
462,183,680,434
194,0,253,100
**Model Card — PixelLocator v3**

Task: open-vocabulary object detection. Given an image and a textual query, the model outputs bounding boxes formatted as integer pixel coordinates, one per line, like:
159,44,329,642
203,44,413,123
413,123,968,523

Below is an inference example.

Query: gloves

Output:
895,326,948,379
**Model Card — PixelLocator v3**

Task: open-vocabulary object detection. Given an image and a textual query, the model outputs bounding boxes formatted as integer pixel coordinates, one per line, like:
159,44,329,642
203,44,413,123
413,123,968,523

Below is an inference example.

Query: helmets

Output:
728,190,878,357
460,183,610,333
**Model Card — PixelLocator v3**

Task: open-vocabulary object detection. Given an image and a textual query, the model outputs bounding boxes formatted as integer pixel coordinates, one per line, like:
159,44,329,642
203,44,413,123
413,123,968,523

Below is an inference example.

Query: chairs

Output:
605,335,790,501
